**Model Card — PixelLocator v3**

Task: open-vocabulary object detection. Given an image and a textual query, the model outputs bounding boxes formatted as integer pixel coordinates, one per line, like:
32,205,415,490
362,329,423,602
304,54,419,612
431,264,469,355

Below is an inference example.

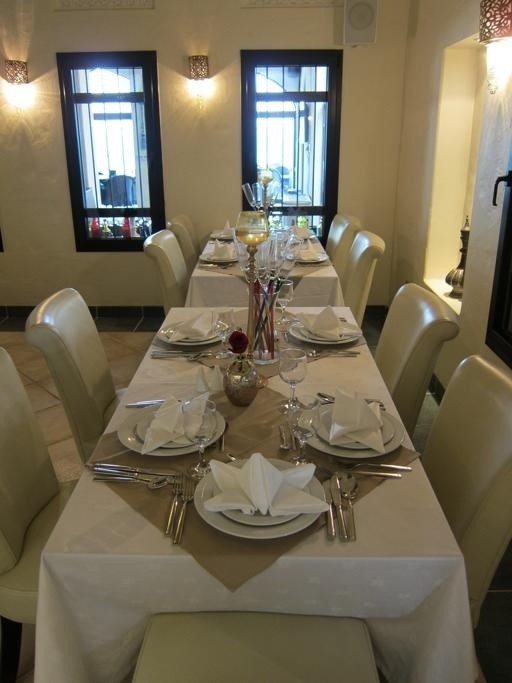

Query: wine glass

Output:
274,348,305,417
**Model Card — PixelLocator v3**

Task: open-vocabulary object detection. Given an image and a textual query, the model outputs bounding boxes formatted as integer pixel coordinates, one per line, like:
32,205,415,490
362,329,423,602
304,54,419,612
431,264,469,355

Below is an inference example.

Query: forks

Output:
174,474,194,545
326,459,412,473
165,470,182,536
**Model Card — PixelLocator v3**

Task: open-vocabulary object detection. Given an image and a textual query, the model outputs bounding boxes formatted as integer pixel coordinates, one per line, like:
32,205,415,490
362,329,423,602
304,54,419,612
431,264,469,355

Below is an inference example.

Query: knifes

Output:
85,462,177,476
318,466,406,479
322,479,335,539
330,471,348,540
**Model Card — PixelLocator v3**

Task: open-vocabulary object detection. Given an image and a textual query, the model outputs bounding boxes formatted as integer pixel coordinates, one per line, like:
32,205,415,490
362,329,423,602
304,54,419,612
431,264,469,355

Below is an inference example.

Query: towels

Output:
200,453,331,521
139,388,213,453
156,307,223,340
295,306,360,339
327,384,385,458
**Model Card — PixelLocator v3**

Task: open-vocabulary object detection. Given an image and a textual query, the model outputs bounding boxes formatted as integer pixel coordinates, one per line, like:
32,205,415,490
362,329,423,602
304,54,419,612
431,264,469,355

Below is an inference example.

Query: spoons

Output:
278,422,293,452
339,475,361,540
93,473,169,489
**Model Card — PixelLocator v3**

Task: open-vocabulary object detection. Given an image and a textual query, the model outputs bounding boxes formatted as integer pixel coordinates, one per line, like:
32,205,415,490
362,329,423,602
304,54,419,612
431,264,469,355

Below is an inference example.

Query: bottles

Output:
83,215,148,237
255,207,326,236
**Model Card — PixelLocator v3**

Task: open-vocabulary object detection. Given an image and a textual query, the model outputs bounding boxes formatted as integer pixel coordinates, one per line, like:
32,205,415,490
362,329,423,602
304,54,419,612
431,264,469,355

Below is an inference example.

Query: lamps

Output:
477,0,512,88
188,54,211,100
2,59,29,107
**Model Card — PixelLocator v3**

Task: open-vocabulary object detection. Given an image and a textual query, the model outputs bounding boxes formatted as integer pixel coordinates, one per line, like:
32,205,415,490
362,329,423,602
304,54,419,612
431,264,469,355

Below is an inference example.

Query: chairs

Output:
371,281,464,445
140,211,385,334
129,608,383,683
1,344,79,588
418,351,512,587
24,283,130,467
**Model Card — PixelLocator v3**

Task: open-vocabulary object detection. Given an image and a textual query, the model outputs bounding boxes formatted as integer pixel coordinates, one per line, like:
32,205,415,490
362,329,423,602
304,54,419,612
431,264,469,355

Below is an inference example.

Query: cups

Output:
312,391,336,415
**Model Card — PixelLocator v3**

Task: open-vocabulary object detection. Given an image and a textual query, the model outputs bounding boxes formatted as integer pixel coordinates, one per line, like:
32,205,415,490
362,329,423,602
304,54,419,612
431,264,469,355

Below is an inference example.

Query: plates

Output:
295,402,405,461
144,227,363,387
193,456,326,544
117,404,228,457
